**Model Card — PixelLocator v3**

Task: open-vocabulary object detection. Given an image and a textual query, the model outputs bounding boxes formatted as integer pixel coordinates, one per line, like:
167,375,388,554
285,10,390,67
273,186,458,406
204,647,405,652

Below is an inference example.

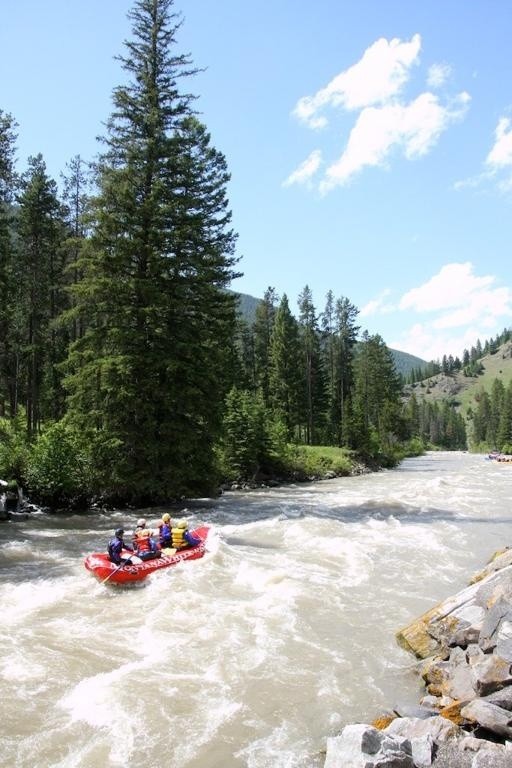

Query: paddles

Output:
100,551,135,583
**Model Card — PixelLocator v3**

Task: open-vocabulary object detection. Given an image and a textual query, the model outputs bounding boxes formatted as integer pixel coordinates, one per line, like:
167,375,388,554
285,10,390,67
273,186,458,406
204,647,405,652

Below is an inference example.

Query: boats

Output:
85,526,209,582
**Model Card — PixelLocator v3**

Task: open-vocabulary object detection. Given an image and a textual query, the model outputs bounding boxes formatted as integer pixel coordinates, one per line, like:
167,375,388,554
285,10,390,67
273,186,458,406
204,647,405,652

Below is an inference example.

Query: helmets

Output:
161,512,170,524
140,530,152,537
115,528,125,538
136,518,146,527
176,519,189,530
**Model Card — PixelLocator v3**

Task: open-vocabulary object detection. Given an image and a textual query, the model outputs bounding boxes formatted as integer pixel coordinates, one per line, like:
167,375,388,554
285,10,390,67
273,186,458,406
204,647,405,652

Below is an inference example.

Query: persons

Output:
137,528,163,562
158,513,173,546
132,518,147,551
109,527,138,565
170,519,204,552
